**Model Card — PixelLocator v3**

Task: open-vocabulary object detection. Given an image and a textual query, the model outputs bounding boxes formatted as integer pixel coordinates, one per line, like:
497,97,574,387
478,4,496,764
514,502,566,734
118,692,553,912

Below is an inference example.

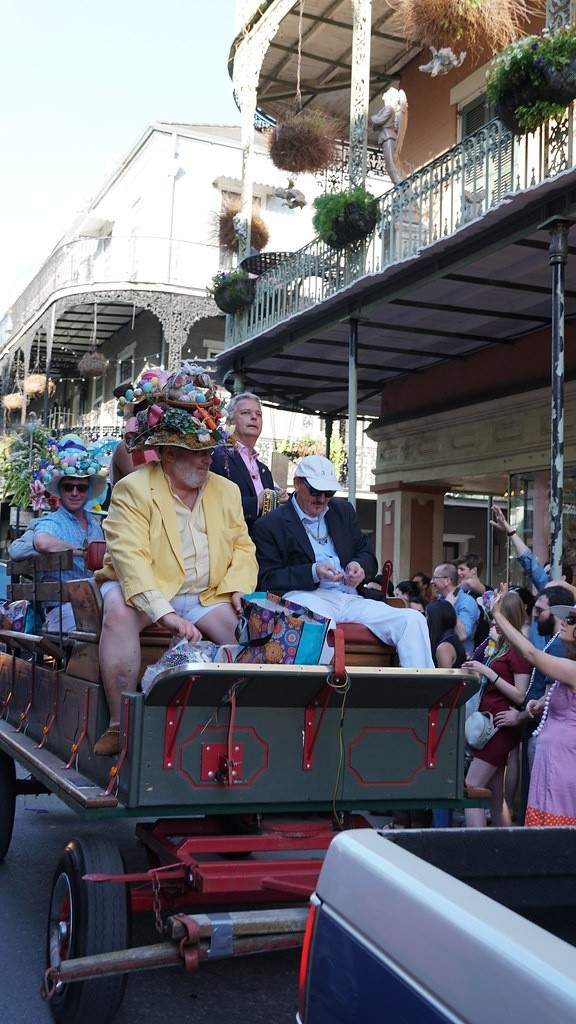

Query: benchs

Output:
0,540,403,681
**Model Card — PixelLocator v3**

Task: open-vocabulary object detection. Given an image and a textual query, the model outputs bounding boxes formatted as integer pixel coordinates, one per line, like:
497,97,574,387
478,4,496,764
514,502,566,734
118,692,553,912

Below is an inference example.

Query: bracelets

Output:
493,675,500,683
507,529,517,536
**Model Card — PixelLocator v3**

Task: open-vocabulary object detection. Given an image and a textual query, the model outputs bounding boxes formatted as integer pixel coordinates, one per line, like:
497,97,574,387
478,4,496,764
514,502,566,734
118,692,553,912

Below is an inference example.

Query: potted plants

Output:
313,184,382,248
78,352,110,377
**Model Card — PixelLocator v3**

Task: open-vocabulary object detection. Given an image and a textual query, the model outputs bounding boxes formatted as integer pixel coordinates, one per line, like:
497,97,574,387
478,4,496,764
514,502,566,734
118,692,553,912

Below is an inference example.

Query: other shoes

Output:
93,723,122,756
380,822,405,831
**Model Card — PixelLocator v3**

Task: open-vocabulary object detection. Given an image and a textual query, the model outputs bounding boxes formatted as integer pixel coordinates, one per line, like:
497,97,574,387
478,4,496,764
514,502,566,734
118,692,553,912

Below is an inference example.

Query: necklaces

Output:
526,629,560,698
532,679,557,736
81,519,87,533
483,639,509,685
303,522,331,544
262,489,279,516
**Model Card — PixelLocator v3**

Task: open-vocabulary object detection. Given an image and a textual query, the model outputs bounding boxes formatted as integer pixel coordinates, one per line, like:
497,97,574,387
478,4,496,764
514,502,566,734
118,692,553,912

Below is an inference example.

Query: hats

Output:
548,602,576,620
294,454,342,492
27,456,57,510
113,361,234,451
42,434,122,504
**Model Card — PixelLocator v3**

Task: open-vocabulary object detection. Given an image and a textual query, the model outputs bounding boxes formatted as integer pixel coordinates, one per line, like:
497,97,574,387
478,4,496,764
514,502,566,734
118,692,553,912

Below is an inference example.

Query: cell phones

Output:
453,586,461,597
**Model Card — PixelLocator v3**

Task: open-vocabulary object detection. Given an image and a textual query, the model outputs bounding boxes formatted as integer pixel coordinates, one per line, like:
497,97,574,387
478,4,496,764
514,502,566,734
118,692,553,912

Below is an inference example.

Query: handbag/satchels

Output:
0,600,44,634
139,637,241,693
233,590,345,676
463,711,494,750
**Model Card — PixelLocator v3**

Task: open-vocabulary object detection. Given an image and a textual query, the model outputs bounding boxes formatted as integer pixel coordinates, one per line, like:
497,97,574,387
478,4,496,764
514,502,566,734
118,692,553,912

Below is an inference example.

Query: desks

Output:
240,252,330,309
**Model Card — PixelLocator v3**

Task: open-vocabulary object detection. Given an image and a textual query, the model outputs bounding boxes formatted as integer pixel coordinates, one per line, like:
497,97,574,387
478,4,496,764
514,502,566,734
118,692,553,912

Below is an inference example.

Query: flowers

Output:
484,19,576,125
484,646,495,658
206,264,249,297
482,590,494,607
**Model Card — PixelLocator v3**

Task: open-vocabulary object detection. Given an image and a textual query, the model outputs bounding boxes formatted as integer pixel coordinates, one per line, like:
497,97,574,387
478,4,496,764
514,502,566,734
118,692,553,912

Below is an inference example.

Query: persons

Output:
371,87,409,184
274,178,307,209
419,45,466,80
252,454,435,669
94,446,259,754
395,504,576,826
376,558,394,595
10,397,159,662
210,390,290,531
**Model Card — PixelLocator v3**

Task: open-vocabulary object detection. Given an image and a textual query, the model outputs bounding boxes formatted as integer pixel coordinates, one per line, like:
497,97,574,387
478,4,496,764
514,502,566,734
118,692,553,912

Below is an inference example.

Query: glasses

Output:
300,480,336,499
564,614,576,624
42,498,62,508
432,574,453,581
171,445,216,456
59,480,90,493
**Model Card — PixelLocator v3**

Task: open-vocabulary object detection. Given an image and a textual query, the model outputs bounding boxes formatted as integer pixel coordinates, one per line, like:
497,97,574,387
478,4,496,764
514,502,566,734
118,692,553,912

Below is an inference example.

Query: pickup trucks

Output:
296,826,576,1024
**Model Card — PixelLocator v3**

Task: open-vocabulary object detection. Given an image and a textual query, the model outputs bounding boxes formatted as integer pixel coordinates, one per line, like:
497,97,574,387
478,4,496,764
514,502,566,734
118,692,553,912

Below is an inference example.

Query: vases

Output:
495,46,576,136
214,279,255,313
